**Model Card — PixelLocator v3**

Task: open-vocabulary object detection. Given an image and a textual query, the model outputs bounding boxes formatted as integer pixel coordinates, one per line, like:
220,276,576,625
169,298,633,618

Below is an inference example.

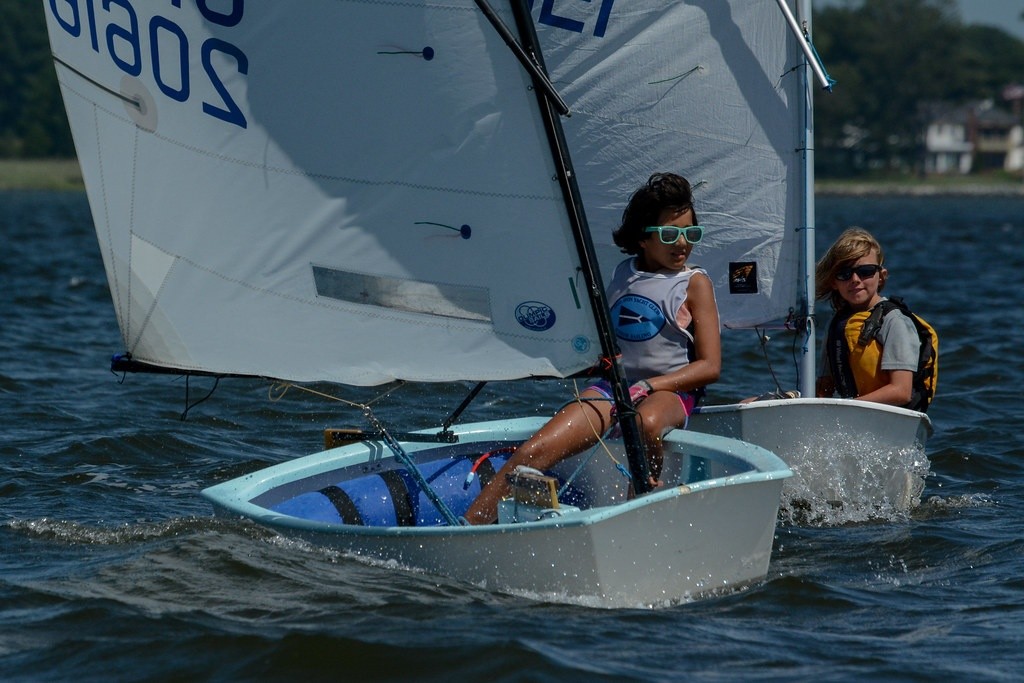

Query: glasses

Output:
835,264,883,282
644,224,706,245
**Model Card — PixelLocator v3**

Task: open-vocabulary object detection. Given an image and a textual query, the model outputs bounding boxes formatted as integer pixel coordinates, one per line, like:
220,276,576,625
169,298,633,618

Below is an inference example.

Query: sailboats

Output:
39,1,796,609
670,1,936,526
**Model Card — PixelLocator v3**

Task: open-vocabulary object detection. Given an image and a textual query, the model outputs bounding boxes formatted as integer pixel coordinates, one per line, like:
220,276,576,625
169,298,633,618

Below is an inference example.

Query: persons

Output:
450,171,723,524
737,227,921,412
